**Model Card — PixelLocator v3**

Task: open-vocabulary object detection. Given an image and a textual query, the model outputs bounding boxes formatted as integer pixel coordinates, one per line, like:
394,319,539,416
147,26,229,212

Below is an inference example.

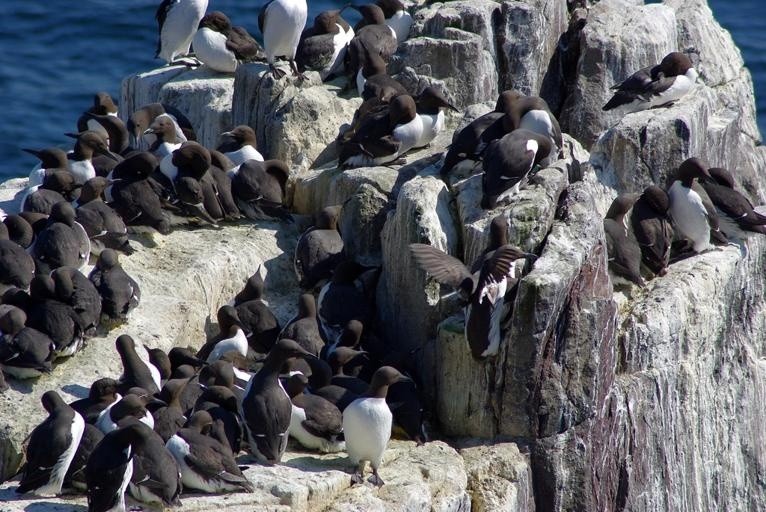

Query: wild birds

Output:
293,196,353,294
439,89,565,210
152,0,413,87
603,157,766,289
407,215,538,363
337,39,460,170
602,51,697,115
1,91,295,381
0,259,433,510
